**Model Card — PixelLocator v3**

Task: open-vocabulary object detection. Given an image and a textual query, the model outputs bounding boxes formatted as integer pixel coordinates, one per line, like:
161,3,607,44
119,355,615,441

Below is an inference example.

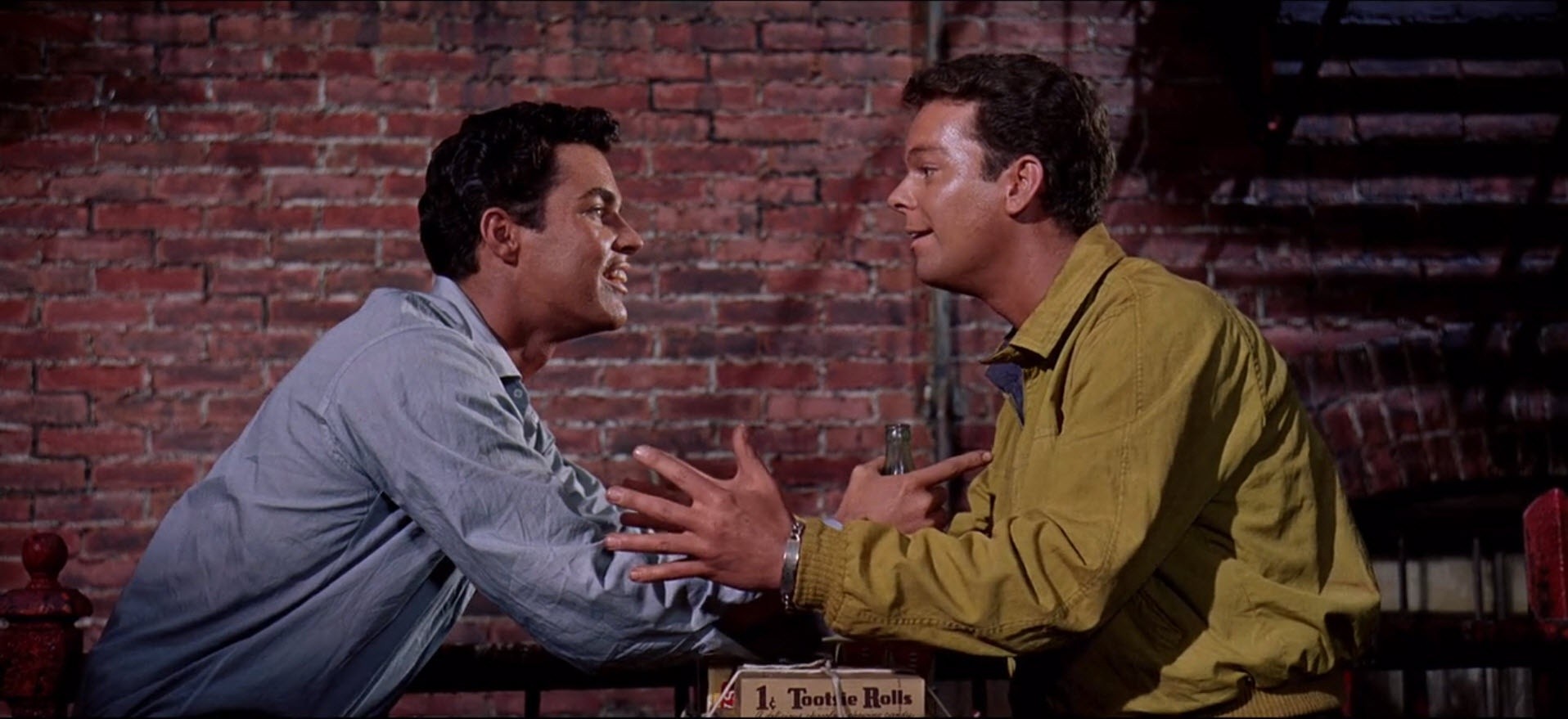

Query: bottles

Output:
881,424,915,476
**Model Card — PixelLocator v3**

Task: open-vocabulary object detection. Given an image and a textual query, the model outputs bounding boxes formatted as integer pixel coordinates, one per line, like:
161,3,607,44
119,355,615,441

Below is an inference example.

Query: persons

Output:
605,54,1383,719
75,100,994,719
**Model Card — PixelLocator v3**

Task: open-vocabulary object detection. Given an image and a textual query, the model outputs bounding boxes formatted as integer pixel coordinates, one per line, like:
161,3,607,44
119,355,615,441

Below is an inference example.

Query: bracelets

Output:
780,522,805,609
